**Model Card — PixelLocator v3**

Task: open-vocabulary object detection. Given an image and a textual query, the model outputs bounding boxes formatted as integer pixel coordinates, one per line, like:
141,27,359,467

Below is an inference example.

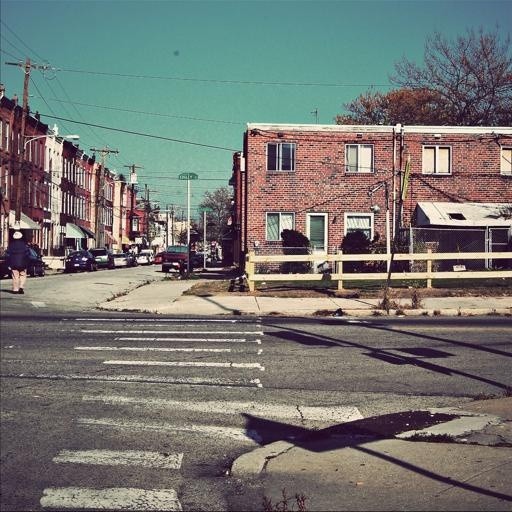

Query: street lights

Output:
15,134,80,223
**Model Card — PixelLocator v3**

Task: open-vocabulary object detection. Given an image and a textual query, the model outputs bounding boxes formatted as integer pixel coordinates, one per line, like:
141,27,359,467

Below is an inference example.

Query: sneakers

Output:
13,288,24,294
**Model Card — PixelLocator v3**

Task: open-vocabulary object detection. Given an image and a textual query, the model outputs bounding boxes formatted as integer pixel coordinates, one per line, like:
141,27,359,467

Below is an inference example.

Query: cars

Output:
64,248,135,272
1,249,45,277
136,252,154,265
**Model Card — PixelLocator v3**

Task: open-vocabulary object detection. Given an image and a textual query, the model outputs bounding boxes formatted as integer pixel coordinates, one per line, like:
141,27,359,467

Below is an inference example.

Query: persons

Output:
5,230,31,294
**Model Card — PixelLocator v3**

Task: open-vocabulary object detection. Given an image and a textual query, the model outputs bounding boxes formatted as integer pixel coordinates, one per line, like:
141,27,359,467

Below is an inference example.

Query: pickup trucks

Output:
161,245,189,272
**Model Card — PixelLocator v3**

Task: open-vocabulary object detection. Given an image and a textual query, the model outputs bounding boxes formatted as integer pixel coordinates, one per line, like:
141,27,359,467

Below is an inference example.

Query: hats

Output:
13,231,22,239
